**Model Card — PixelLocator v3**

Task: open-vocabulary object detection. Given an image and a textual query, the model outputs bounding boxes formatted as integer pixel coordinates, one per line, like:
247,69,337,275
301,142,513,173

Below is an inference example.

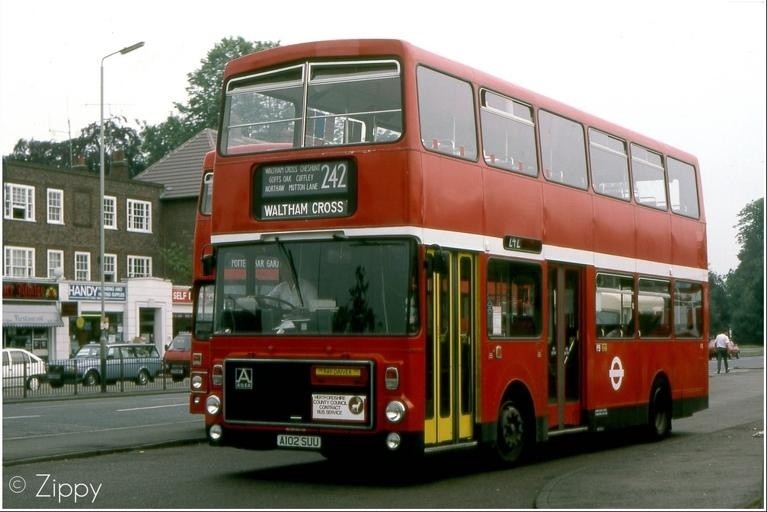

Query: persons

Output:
714,329,730,374
257,258,318,312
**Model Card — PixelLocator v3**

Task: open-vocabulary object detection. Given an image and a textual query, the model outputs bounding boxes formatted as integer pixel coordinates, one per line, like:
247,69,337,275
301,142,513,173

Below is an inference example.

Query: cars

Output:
710,338,741,360
3,334,191,396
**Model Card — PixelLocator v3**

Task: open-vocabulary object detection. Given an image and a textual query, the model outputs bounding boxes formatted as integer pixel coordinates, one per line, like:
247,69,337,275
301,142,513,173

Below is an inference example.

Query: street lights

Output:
99,41,145,389
